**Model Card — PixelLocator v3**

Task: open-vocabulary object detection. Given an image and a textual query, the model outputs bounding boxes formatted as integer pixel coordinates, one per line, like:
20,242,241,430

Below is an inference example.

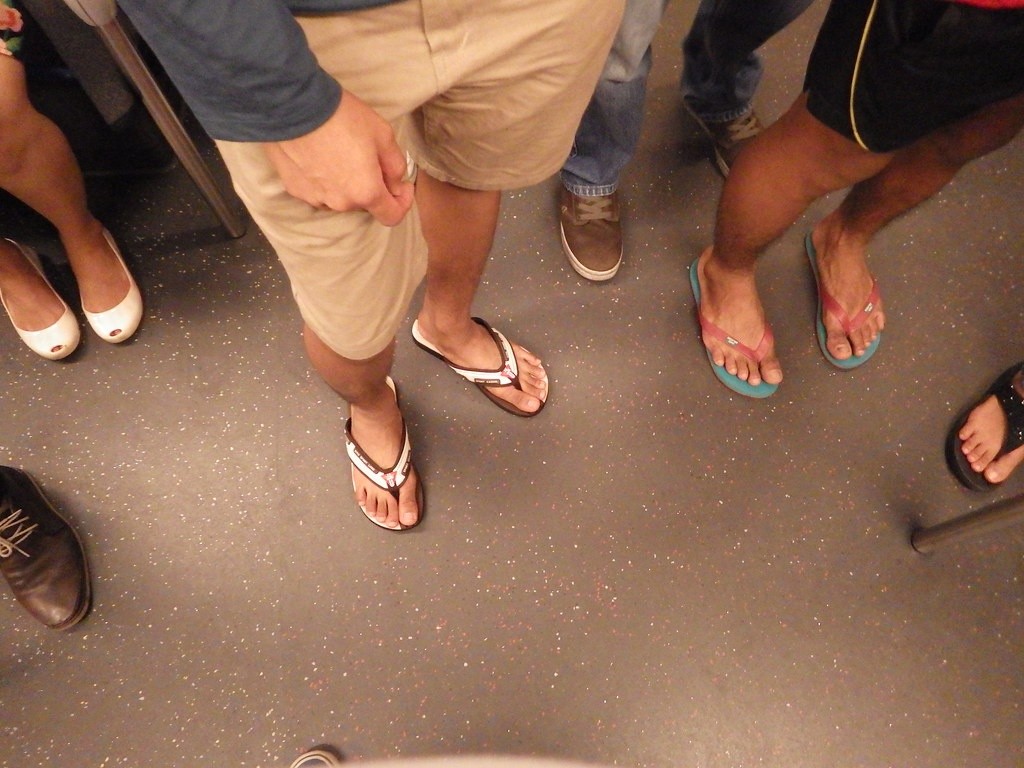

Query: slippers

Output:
805,232,880,370
412,320,548,417
947,362,1024,492
690,259,779,399
338,376,424,531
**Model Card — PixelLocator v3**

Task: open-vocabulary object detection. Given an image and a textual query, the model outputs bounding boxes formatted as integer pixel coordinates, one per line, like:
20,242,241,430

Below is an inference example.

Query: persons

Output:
112,0,629,532
945,360,1024,494
691,1,1023,398
559,1,820,281
0,464,90,632
0,0,144,361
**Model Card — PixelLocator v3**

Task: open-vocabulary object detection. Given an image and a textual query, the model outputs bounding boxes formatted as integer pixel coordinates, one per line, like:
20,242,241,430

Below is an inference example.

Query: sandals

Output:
0,238,78,361
80,227,143,344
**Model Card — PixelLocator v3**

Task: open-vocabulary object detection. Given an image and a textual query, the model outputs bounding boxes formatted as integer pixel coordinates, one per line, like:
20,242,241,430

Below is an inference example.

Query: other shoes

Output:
290,751,339,768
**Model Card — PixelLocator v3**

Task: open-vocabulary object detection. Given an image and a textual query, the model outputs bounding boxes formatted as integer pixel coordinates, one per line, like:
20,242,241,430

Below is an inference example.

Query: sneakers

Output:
685,103,765,179
558,185,622,281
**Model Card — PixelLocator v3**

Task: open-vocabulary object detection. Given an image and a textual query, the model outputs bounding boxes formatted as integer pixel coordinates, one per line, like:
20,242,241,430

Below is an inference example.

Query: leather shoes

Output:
0,465,89,631
